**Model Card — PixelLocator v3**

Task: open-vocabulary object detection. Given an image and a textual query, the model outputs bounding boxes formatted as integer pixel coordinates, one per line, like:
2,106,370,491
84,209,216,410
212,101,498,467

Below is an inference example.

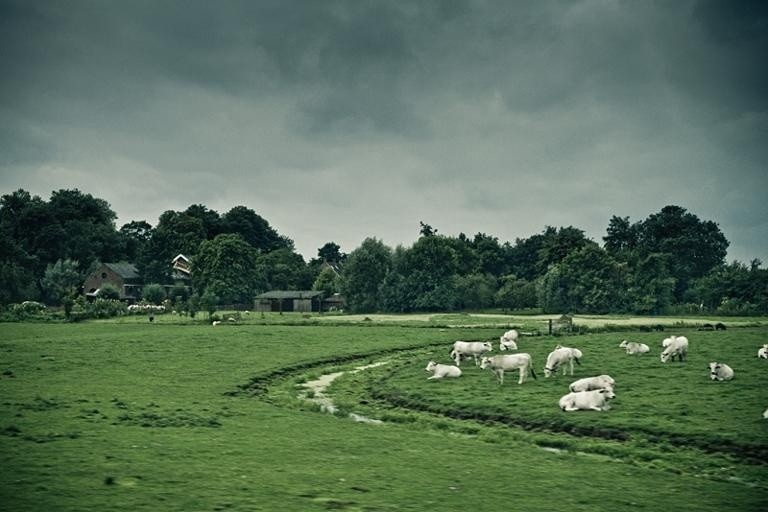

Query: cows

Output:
660,335,690,364
761,409,768,419
424,357,462,382
448,341,494,367
618,339,650,356
499,329,518,351
542,344,583,379
706,360,734,382
758,344,768,360
479,353,538,385
559,374,616,412
